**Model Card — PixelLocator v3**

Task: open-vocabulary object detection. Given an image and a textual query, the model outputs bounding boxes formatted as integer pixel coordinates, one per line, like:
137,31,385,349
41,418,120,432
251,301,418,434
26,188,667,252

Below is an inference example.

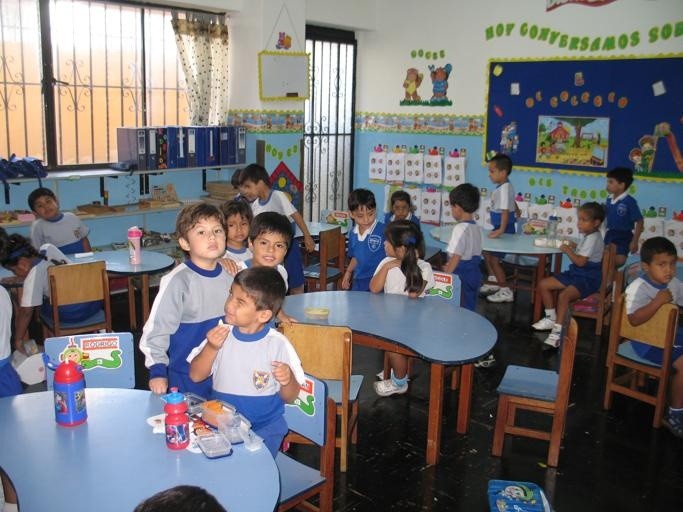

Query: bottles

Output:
54,360,88,427
545,214,558,248
128,226,142,265
165,386,190,450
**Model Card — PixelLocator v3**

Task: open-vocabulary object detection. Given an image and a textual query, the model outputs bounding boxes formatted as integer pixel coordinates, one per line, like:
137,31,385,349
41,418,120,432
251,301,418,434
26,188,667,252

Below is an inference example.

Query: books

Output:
116,125,246,172
199,182,240,202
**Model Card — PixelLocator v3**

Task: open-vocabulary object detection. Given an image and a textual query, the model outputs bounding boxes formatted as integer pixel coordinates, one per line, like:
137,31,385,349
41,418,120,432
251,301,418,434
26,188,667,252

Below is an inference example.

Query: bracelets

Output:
345,269,352,274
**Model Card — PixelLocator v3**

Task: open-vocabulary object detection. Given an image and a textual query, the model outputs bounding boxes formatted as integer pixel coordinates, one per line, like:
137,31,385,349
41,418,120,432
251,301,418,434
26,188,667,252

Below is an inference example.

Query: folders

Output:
116,126,158,172
166,126,246,169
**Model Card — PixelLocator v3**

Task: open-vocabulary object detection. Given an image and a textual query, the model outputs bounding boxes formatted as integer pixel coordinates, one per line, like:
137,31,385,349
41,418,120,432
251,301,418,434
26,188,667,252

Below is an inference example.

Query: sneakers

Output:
480,283,499,296
486,287,514,303
374,367,408,397
531,317,553,330
474,354,496,369
662,407,682,437
544,329,560,348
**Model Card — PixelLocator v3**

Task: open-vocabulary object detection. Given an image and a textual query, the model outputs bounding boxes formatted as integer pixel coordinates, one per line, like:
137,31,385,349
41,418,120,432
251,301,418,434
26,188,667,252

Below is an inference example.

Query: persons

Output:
368,223,434,397
603,167,644,287
238,212,298,294
0,284,12,360
26,187,98,253
339,189,391,292
237,165,319,294
627,241,682,440
382,189,423,224
488,152,523,305
0,233,106,357
441,185,486,310
138,203,241,400
217,197,252,276
225,164,241,196
185,267,306,459
531,200,605,350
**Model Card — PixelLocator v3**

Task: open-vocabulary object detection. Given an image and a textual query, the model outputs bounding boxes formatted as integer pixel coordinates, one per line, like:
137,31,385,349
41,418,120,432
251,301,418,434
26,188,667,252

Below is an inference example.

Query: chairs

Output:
299,225,348,296
573,244,678,428
43,331,137,389
499,217,558,306
491,317,578,467
37,259,115,341
275,372,335,512
278,318,366,472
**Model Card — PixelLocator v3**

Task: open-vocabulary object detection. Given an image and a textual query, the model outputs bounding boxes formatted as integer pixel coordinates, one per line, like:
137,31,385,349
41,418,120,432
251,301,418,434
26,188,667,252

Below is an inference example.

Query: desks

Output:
2,387,282,512
294,221,347,245
0,246,175,334
427,226,581,325
279,290,499,468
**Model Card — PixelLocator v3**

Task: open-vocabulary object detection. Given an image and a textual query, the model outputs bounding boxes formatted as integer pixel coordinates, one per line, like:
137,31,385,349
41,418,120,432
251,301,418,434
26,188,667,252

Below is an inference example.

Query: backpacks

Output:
0,157,18,179
8,154,47,178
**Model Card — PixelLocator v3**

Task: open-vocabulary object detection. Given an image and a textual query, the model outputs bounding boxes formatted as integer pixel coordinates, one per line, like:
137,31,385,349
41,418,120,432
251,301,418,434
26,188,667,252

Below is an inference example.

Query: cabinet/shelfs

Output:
0,163,249,296
